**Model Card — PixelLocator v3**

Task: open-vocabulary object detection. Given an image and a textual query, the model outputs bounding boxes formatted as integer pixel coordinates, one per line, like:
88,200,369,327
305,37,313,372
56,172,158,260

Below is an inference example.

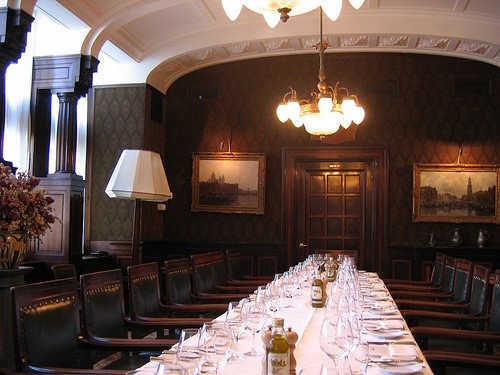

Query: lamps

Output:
104,149,173,266
221,0,364,30
276,7,366,141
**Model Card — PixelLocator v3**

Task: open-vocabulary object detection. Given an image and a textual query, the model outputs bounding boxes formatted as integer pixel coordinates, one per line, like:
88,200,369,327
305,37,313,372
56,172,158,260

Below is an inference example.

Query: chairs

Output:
315,249,357,273
0,248,285,375
379,251,500,375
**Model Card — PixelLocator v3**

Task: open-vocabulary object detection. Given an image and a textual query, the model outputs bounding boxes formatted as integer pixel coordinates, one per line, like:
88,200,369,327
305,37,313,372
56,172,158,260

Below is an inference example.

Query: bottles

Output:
311,267,323,308
265,317,290,375
327,257,335,281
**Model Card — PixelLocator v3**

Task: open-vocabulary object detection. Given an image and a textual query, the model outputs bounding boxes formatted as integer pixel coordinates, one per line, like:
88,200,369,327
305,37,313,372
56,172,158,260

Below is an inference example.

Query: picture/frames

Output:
412,163,500,225
190,151,267,215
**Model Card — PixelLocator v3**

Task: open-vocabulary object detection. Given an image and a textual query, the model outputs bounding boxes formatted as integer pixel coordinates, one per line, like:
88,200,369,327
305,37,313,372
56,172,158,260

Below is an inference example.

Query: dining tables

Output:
134,270,435,375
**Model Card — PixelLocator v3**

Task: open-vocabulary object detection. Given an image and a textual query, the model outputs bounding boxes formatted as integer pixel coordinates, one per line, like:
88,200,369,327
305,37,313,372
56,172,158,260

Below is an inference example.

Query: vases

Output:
0,228,35,269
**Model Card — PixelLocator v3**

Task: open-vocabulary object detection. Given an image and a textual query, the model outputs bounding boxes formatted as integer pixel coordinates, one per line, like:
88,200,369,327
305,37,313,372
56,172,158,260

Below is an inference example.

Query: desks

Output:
108,242,284,281
0,265,34,375
82,255,114,274
386,244,500,307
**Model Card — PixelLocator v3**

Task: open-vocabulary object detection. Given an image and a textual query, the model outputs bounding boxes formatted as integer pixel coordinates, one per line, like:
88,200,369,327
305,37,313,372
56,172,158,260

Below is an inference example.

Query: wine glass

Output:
163,253,369,375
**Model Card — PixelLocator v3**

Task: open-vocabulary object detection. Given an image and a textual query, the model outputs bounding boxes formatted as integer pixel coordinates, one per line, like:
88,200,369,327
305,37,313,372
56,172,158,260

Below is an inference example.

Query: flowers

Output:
0,162,64,243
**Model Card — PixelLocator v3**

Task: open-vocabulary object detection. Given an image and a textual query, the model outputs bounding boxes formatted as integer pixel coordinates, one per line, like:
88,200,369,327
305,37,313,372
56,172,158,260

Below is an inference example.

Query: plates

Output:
359,269,423,375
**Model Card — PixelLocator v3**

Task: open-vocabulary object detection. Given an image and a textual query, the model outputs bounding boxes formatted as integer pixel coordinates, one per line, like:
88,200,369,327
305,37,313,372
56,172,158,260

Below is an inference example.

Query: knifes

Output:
363,317,402,321
367,340,415,345
371,359,423,363
